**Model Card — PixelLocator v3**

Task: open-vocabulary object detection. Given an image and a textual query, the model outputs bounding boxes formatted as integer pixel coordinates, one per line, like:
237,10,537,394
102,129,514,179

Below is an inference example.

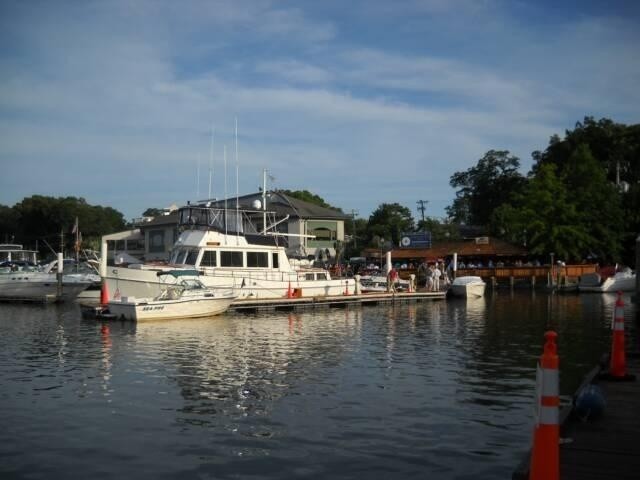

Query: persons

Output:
457,259,542,267
432,266,442,291
423,265,434,292
388,267,398,293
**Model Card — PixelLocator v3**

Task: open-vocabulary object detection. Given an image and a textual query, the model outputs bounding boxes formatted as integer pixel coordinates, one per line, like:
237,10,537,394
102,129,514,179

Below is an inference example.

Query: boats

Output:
360,273,411,292
0,244,100,303
546,267,635,293
451,273,487,300
109,280,236,322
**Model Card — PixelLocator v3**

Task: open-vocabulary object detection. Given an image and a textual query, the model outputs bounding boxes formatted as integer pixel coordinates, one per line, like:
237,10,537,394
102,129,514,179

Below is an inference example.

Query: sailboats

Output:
107,118,362,299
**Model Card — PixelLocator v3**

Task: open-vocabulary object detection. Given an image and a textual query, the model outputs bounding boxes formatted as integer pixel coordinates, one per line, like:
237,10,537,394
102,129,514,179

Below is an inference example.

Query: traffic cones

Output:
528,331,559,480
601,291,635,380
